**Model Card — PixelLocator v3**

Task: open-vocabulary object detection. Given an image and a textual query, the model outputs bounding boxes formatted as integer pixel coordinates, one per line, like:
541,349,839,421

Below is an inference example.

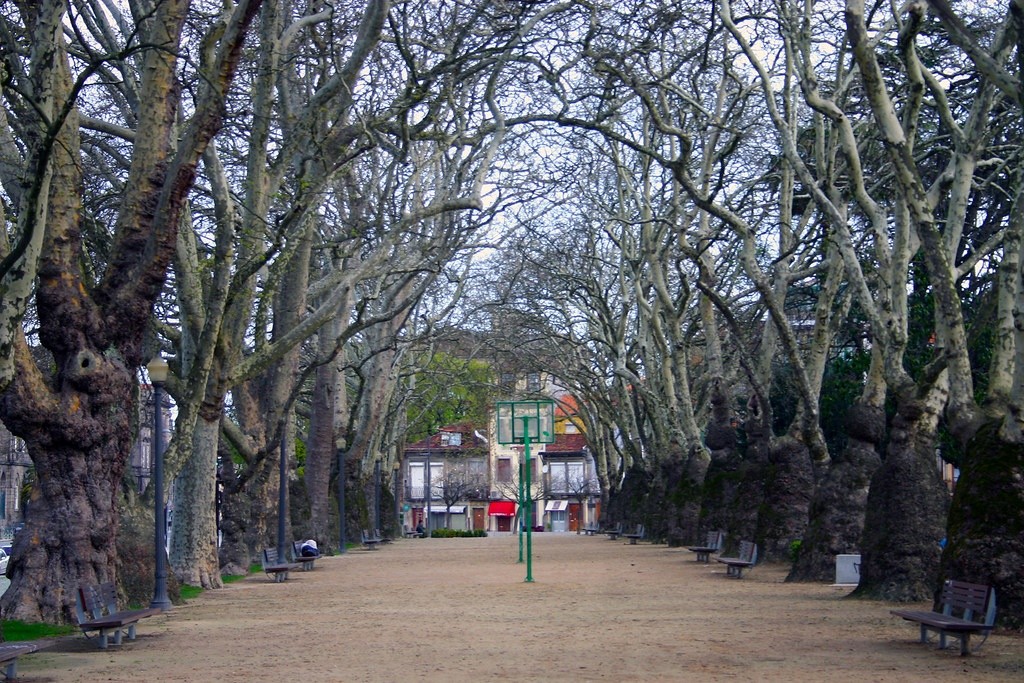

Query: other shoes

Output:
305,551,311,556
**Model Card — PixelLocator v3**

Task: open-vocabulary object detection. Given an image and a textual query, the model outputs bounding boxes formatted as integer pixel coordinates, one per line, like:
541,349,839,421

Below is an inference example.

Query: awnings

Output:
424,506,466,514
488,501,515,516
545,500,568,512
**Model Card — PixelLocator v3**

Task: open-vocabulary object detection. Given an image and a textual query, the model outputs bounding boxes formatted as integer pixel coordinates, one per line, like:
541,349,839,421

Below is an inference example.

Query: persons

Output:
417,520,424,533
301,539,319,557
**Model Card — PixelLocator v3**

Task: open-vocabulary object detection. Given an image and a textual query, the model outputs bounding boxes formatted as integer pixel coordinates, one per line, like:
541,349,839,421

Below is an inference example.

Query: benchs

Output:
291,540,322,571
622,524,644,545
688,532,721,563
411,528,423,538
374,529,392,546
262,547,303,583
890,576,1000,657
75,582,161,648
362,529,383,550
403,525,417,537
715,540,758,579
0,636,55,683
581,521,600,535
605,522,622,540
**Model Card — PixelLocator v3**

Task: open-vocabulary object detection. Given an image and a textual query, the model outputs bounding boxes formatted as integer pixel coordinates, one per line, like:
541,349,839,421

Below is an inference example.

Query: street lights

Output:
392,461,400,538
373,450,383,546
145,354,173,611
275,406,290,583
335,436,346,555
